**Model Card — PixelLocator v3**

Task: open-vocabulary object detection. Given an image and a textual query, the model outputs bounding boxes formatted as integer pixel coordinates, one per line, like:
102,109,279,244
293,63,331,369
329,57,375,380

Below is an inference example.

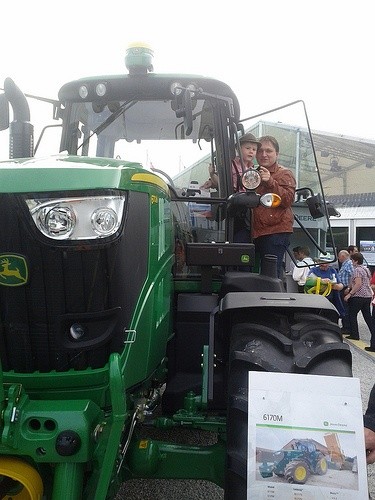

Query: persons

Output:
363,383,375,464
201,133,296,282
290,243,375,354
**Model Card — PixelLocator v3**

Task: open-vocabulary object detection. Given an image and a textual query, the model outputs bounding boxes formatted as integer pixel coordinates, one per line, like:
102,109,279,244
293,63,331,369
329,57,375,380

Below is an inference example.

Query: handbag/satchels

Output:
304,277,334,296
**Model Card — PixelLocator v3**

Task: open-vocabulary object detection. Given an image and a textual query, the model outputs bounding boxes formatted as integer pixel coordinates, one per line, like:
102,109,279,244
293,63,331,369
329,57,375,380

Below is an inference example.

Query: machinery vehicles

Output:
259,439,330,484
0,44,352,499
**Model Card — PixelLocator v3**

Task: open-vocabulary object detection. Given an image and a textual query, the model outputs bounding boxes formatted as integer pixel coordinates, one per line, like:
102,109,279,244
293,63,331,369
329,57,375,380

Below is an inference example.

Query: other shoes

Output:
345,336,359,340
365,347,375,352
340,327,350,334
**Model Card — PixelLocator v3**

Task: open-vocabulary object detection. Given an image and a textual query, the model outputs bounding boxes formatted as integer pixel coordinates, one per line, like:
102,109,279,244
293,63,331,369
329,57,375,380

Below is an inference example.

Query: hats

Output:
235,133,262,150
314,252,335,262
